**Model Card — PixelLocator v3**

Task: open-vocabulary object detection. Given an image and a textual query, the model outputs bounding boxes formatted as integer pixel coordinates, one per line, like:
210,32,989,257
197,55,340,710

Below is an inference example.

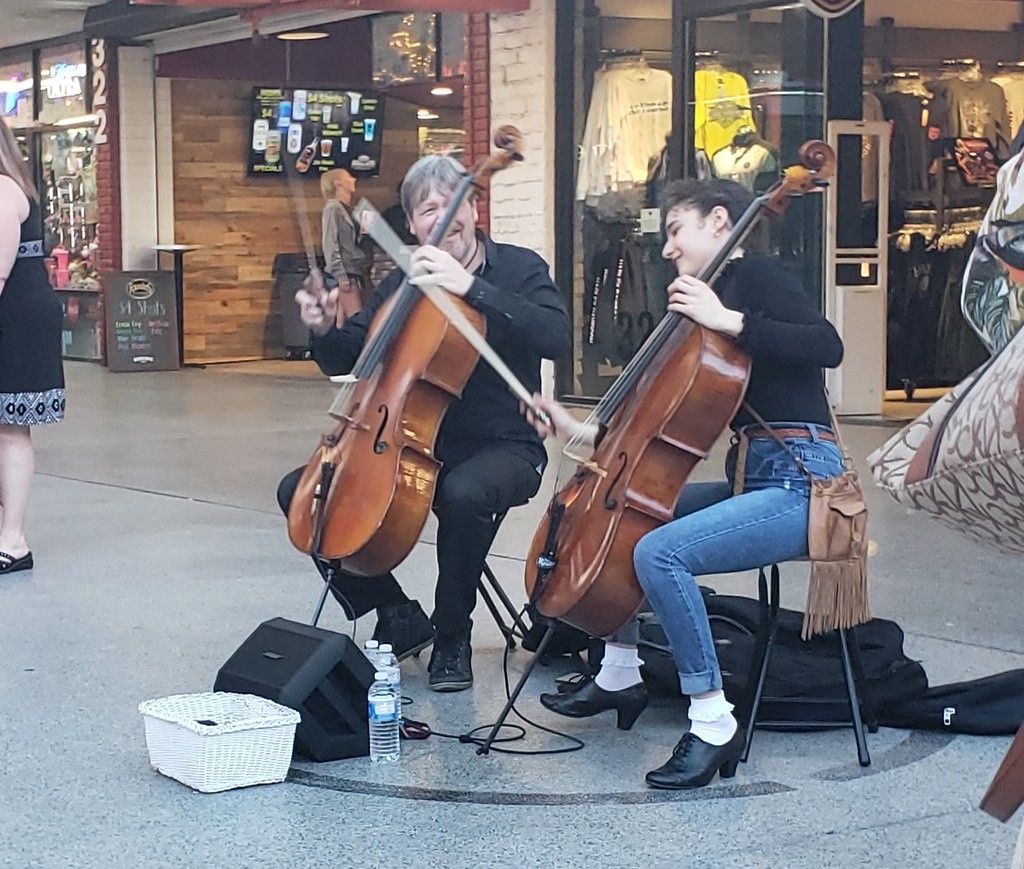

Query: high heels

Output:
539,671,648,730
646,720,745,789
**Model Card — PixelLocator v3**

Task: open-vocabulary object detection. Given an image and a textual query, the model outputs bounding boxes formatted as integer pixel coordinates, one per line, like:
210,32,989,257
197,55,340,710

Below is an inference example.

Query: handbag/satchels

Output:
801,470,871,640
865,318,1024,557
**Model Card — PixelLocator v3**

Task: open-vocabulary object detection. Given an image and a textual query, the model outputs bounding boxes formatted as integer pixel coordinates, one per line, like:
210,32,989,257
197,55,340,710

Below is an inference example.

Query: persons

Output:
519,178,848,790
647,124,782,196
361,179,420,267
277,154,572,691
322,169,365,291
0,117,66,574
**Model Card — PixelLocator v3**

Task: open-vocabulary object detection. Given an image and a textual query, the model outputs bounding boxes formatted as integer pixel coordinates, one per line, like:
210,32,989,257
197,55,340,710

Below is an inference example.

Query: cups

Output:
322,109,332,122
341,138,349,152
292,89,307,120
349,92,362,115
321,140,333,157
363,119,376,141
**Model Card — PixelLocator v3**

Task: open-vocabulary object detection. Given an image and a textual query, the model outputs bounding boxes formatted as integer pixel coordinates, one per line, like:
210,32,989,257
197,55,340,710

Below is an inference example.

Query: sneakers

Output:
365,600,435,664
426,613,473,690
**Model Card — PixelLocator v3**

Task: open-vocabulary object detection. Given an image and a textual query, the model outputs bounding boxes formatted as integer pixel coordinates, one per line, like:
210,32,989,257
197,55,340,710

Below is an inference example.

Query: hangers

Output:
895,208,989,247
887,59,982,97
597,48,651,76
689,51,726,74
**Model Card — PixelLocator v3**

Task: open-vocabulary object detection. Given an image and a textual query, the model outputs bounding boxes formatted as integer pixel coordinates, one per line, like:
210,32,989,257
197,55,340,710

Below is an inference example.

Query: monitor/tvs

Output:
247,86,386,177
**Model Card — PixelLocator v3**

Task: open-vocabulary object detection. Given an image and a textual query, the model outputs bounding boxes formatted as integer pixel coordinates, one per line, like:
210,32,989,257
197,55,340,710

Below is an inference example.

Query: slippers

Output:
0,551,34,574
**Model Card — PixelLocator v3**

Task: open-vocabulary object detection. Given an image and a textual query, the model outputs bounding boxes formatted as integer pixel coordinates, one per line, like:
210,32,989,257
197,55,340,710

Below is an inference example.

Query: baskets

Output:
138,692,301,793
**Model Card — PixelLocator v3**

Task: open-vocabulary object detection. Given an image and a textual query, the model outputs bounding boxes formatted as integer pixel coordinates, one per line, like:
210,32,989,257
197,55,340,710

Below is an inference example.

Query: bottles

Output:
375,643,401,719
295,137,319,173
364,640,379,668
368,671,400,762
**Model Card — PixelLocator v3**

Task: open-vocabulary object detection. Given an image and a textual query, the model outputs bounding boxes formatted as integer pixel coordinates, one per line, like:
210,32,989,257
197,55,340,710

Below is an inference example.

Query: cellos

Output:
284,121,527,629
474,135,839,762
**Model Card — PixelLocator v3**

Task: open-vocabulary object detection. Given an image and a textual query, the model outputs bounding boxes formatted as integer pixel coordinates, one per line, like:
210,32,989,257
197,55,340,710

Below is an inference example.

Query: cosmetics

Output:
250,90,307,162
364,118,376,141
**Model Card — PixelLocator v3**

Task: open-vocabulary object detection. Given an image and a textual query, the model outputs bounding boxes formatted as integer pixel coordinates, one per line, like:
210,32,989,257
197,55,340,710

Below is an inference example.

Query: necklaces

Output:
464,238,479,269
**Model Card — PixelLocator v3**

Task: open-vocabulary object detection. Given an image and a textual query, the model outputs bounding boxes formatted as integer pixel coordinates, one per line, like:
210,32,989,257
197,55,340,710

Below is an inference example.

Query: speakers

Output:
212,619,407,765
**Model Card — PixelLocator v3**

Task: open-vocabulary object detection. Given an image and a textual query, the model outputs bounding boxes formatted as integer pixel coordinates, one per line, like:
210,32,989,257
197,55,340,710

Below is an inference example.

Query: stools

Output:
479,498,531,646
734,554,881,769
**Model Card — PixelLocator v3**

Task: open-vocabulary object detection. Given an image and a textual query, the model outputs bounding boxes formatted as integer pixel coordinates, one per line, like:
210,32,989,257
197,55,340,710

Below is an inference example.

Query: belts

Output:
732,428,835,496
